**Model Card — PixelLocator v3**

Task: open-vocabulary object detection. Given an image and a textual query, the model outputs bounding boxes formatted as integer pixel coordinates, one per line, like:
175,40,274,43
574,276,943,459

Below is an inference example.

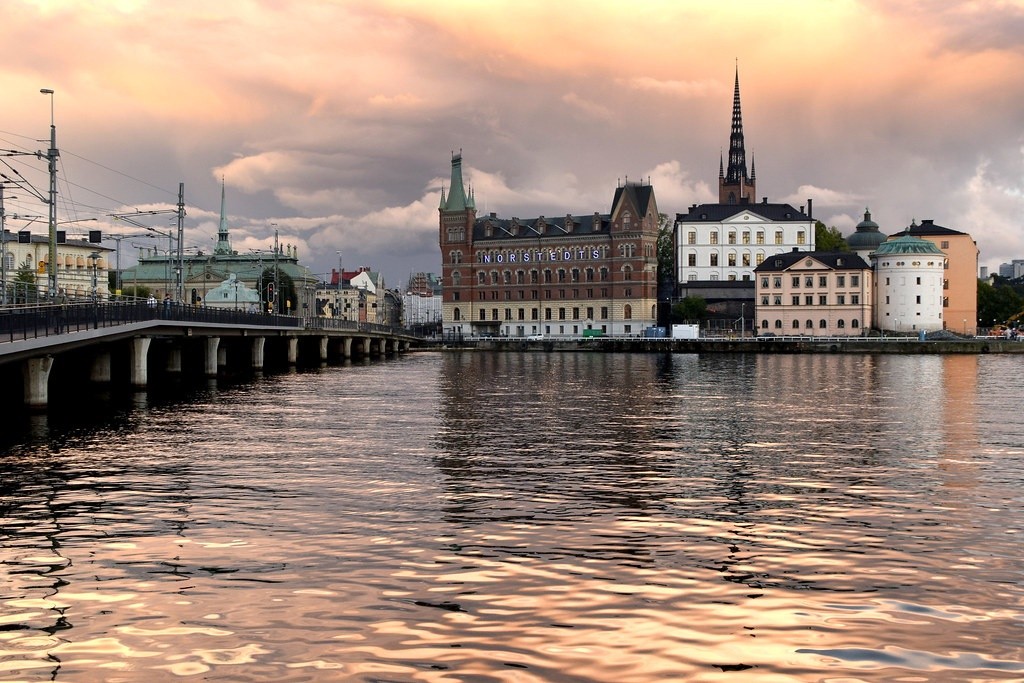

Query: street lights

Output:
54,218,98,295
337,250,342,321
304,266,309,287
740,303,745,342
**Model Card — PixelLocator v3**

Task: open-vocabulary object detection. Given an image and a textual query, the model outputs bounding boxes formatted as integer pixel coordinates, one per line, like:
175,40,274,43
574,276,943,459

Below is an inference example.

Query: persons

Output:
163,292,173,309
146,293,158,309
53,287,69,334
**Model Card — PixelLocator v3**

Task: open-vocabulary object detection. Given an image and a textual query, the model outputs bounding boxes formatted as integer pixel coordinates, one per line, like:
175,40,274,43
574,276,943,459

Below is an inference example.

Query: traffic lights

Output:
268,283,275,304
192,288,196,304
